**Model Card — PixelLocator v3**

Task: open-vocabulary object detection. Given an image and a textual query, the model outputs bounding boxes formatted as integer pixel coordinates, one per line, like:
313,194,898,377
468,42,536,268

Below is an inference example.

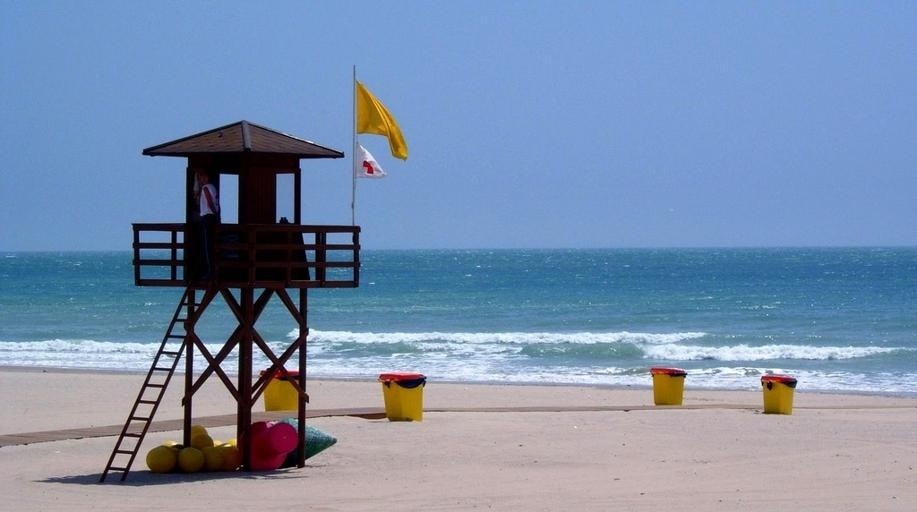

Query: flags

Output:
355,78,408,159
355,142,386,179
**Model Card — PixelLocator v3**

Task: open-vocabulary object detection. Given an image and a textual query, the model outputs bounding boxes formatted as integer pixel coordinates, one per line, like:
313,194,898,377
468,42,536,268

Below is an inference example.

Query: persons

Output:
197,166,220,274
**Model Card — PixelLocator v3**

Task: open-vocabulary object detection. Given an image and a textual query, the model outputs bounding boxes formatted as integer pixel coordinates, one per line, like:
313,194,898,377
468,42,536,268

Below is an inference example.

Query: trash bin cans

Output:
257,369,299,412
377,371,427,421
649,367,688,405
760,376,798,415
